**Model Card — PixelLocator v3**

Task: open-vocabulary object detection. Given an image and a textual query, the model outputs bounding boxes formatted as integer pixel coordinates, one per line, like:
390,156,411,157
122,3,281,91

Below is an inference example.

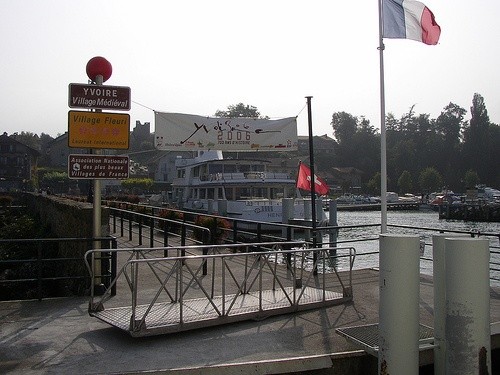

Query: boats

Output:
318,183,500,211
158,147,329,234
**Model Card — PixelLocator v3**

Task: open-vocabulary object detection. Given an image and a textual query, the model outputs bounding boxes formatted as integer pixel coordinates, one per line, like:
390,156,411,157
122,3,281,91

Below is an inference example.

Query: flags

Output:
296,160,330,196
379,0,441,46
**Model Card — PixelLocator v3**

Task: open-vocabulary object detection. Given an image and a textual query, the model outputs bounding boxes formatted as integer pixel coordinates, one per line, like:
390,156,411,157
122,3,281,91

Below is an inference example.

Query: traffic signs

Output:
67,109,130,150
67,153,130,181
67,82,132,112
